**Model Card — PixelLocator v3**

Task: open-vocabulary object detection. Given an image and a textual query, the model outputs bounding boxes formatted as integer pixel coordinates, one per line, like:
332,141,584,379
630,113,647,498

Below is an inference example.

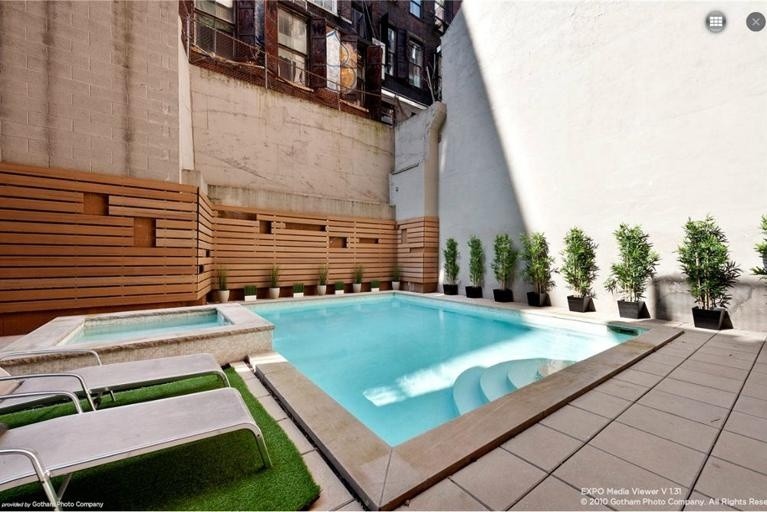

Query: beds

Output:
1,347,274,511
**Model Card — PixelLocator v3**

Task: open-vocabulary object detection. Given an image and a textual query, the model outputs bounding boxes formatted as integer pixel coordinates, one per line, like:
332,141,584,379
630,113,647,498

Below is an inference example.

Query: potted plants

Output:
209,264,406,304
441,220,660,319
670,212,741,331
